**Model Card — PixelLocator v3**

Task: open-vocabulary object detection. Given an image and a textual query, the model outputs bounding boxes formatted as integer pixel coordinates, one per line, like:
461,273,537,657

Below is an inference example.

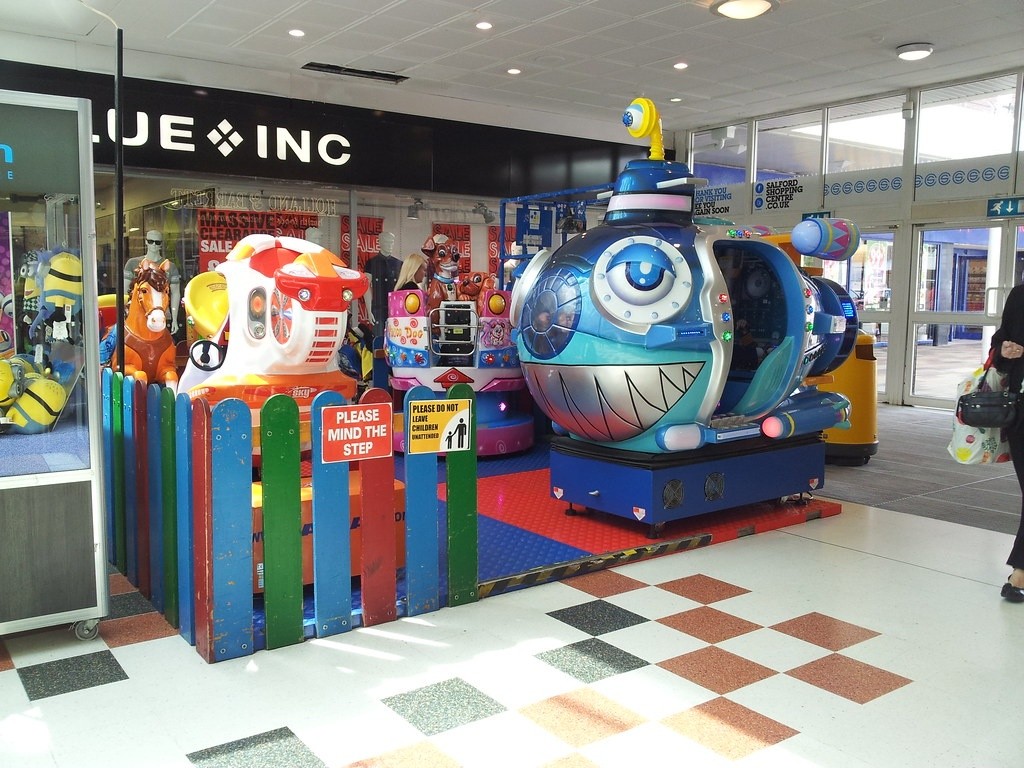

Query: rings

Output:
1017,349,1020,352
1013,352,1016,353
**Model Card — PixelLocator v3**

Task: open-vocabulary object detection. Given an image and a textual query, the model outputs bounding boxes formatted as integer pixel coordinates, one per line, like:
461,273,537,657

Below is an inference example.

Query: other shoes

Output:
1001,583,1024,602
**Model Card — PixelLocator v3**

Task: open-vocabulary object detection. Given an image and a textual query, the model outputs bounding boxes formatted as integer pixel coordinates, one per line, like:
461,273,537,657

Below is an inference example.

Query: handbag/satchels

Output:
956,342,1016,428
948,346,1013,465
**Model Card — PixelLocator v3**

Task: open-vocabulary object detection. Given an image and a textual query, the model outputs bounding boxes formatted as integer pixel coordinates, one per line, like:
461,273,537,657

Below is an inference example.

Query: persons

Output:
393,253,428,289
305,227,321,247
989,282,1023,602
363,230,405,338
123,228,181,336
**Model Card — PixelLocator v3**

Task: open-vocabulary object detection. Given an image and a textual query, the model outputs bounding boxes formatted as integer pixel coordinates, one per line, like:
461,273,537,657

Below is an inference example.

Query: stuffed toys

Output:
18,249,46,311
29,246,84,345
0,358,76,435
0,350,52,410
